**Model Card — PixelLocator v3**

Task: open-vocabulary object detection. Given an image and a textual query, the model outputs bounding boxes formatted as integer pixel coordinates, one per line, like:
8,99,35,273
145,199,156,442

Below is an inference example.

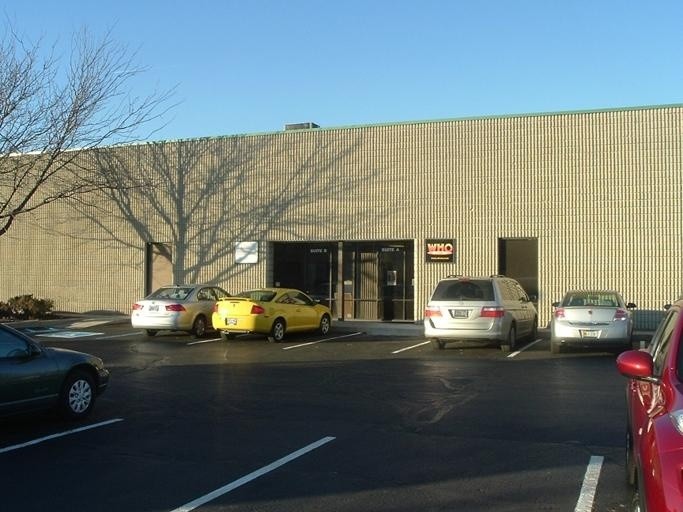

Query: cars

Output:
549,290,637,352
617,299,682,512
129,284,232,339
0,322,109,418
212,288,333,343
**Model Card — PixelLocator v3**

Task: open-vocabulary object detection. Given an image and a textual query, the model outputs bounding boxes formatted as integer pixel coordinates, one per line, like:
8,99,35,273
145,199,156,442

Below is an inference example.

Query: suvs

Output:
424,274,538,352
